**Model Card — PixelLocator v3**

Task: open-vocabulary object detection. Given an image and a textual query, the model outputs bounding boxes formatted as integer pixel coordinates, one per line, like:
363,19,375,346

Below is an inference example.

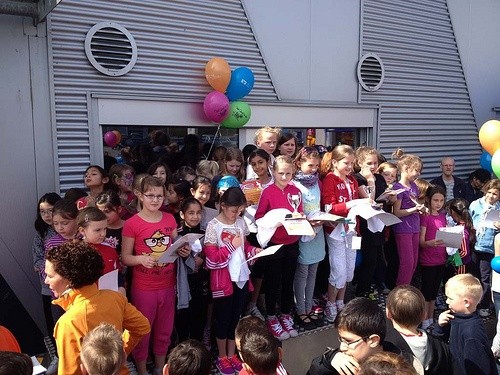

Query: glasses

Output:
39,210,54,215
337,333,372,346
141,191,164,200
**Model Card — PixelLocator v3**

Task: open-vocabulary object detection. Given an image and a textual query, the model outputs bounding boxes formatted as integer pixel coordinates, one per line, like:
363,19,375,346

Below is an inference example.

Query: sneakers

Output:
246,307,265,322
312,299,322,313
267,314,298,340
201,326,213,351
324,298,346,323
214,354,243,375
424,323,446,337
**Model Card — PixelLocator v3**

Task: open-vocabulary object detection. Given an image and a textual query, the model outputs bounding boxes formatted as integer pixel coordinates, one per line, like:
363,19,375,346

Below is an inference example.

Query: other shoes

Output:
296,312,323,329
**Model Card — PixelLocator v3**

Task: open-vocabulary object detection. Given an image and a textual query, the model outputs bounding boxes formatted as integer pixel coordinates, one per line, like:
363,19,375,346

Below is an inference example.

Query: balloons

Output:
491,150,500,179
480,152,493,173
104,131,117,147
204,91,230,123
221,101,251,128
225,67,254,101
112,130,121,144
205,58,231,93
479,120,500,156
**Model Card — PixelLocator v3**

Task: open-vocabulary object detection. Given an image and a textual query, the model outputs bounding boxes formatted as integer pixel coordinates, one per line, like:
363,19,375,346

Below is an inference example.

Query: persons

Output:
0,144,500,375
104,130,228,173
273,134,297,180
246,127,281,179
